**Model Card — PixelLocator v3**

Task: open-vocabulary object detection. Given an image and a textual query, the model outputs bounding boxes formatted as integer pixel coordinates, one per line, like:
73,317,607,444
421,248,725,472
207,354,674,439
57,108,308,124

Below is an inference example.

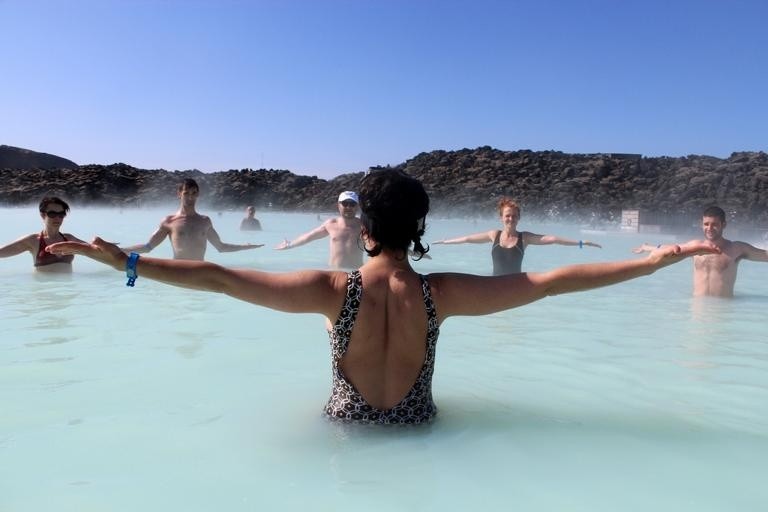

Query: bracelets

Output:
125,252,139,287
579,240,582,249
287,240,292,249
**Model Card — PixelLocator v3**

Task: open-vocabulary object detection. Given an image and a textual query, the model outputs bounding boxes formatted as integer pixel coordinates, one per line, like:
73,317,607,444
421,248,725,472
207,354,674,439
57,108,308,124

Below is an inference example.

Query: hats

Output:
338,190,359,204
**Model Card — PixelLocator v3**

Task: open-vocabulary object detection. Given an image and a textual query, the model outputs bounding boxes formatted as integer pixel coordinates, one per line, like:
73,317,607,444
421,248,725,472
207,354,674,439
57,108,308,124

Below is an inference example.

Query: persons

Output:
117,177,267,267
631,205,768,301
430,198,603,277
274,190,433,271
0,194,124,277
237,206,262,232
41,165,725,426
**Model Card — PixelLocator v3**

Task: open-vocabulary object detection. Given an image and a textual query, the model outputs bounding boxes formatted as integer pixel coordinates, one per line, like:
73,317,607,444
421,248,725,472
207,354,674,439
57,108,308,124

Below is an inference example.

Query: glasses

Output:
339,201,357,207
42,210,66,219
181,191,199,197
